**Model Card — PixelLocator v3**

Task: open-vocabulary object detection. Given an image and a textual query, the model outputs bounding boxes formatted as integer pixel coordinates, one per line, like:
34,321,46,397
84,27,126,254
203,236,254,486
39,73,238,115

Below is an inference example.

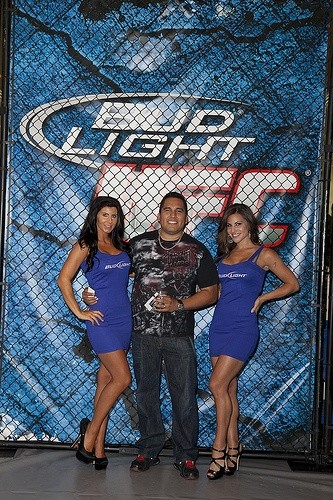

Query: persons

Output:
83,191,220,481
56,195,138,470
207,202,301,481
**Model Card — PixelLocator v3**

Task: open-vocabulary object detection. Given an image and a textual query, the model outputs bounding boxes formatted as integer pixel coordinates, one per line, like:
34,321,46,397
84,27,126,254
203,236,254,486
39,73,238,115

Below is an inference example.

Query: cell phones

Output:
87,287,95,298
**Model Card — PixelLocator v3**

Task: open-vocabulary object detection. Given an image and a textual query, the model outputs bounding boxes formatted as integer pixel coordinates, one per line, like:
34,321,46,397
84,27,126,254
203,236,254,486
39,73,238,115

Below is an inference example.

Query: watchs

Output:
177,299,186,313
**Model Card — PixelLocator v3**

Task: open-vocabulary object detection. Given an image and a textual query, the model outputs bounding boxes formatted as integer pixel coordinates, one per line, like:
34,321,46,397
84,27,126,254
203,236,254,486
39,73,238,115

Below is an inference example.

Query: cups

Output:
155,290,168,309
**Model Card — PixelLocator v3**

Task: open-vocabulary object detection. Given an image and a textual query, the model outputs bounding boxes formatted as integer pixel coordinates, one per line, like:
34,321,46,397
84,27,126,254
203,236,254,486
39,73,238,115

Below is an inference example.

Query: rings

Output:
164,305,167,308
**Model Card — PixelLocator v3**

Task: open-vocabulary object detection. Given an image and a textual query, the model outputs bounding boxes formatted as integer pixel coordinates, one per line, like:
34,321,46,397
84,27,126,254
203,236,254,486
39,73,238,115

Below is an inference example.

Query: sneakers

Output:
173,456,199,480
130,455,160,472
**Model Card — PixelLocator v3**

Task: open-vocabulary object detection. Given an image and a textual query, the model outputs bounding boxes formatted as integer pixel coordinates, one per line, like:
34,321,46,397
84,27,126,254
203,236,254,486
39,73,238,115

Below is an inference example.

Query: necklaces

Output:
159,227,184,251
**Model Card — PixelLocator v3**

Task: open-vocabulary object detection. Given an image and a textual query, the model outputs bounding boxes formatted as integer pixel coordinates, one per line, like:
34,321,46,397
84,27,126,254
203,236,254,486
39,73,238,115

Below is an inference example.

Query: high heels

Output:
223,443,243,476
70,418,94,464
207,444,227,480
94,451,108,471
